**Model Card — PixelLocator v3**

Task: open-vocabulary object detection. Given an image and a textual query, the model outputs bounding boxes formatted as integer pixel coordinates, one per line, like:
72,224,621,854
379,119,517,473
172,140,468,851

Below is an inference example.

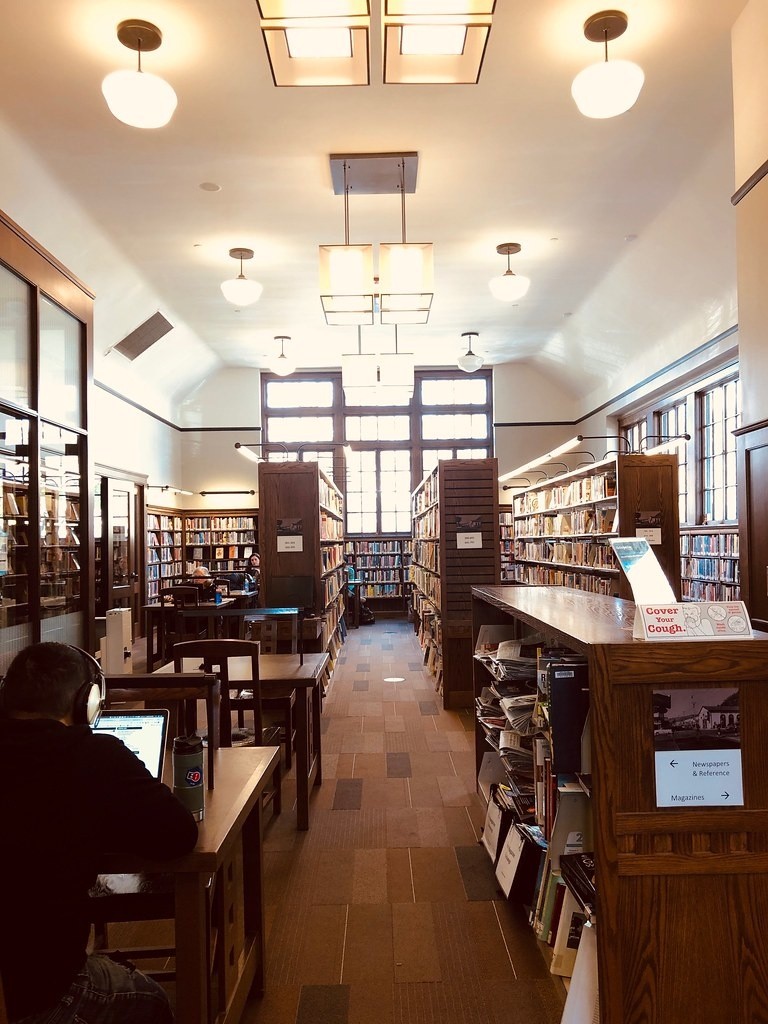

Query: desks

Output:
151,654,329,831
219,589,259,637
140,598,235,673
88,746,280,1024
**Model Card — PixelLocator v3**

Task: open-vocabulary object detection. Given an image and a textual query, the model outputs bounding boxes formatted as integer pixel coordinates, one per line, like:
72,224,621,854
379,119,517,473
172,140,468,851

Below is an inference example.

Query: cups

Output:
214,593,221,603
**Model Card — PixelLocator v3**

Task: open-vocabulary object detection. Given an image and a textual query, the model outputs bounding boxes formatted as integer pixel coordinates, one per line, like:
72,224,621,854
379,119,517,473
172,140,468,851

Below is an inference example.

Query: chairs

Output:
94,579,299,1011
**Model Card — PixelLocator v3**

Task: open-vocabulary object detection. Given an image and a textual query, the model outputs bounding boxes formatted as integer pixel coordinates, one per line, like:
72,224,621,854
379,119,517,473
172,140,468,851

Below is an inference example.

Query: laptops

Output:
90,709,170,785
173,583,203,604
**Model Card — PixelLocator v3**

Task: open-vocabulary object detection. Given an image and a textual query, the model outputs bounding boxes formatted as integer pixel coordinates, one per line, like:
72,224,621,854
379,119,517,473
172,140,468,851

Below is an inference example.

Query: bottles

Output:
244,579,249,591
172,735,205,822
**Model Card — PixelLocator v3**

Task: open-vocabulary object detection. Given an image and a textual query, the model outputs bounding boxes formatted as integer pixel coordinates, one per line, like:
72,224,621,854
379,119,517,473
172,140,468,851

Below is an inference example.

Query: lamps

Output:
269,335,296,375
572,11,646,118
99,19,178,127
258,0,496,88
220,247,262,307
456,331,484,372
164,433,691,496
315,151,434,326
488,242,530,303
340,325,413,407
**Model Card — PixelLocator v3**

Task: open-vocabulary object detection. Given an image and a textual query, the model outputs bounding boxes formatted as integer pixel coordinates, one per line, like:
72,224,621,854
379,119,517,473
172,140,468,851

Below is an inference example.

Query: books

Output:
0,491,80,625
409,474,443,696
680,534,740,602
345,541,413,596
318,478,348,687
148,515,257,604
475,625,599,1024
498,470,620,599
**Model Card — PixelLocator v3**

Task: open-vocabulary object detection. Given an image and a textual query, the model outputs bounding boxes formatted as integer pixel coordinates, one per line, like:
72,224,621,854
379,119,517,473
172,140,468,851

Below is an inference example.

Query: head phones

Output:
0,643,106,725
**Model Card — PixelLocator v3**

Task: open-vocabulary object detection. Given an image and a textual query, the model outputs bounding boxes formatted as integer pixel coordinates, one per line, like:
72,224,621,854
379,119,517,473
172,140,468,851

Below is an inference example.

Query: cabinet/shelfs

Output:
147,453,739,711
0,479,80,623
470,585,768,1024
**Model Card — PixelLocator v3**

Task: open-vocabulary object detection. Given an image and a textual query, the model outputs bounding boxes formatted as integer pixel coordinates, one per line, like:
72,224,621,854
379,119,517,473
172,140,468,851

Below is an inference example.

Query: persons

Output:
344,555,366,603
246,553,260,575
116,556,127,585
164,567,218,602
0,642,197,1024
